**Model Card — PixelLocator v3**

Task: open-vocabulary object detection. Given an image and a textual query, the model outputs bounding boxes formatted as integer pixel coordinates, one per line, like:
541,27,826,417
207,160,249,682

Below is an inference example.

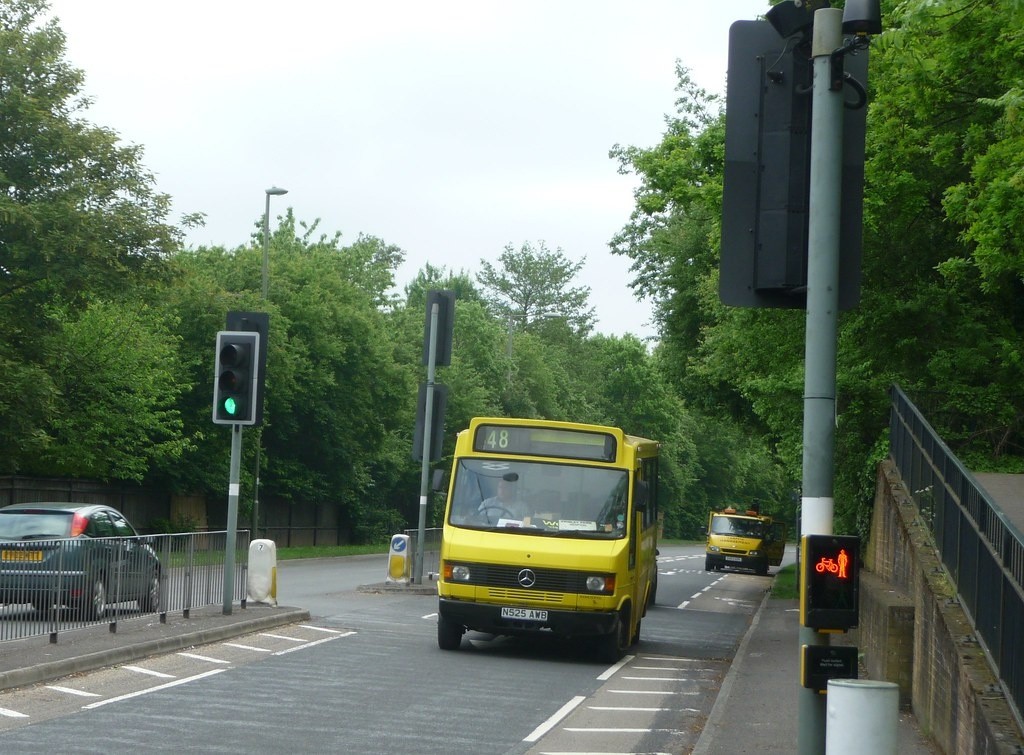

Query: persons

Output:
746,522,769,539
477,479,531,520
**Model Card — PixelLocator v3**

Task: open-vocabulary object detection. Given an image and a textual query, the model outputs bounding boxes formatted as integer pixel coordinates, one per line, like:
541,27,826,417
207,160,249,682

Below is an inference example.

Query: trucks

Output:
706,506,788,576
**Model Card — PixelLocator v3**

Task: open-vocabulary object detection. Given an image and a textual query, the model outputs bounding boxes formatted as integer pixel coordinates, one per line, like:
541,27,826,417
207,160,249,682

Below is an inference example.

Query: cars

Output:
1,500,164,616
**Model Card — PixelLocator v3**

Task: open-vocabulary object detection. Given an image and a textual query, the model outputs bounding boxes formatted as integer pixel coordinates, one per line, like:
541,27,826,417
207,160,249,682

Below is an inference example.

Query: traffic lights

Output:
800,535,860,631
212,330,263,426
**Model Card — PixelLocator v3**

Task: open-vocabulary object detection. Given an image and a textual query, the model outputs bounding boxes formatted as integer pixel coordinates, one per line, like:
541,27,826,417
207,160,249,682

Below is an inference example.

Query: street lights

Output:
250,185,288,548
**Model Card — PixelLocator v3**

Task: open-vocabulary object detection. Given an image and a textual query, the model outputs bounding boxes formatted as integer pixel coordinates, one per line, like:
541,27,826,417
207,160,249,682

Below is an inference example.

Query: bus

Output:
429,415,662,662
